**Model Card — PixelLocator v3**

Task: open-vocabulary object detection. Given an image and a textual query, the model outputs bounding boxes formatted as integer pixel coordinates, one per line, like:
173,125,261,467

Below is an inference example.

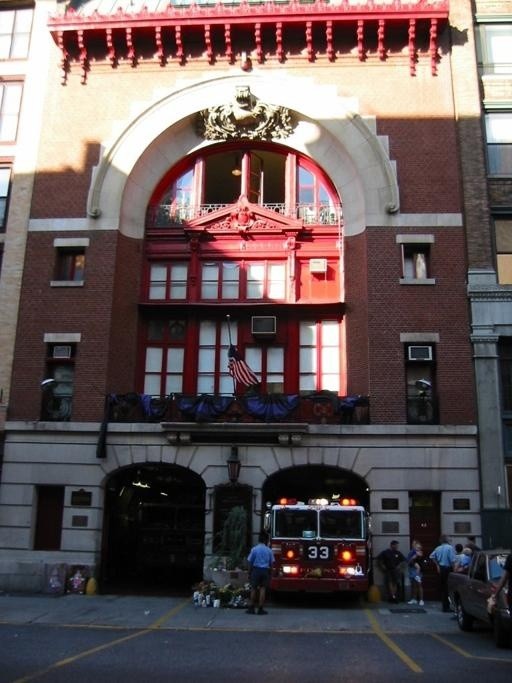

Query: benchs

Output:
227,341,259,390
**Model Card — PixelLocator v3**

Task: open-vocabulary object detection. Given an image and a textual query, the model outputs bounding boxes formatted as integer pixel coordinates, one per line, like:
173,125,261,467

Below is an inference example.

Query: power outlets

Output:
444,547,511,649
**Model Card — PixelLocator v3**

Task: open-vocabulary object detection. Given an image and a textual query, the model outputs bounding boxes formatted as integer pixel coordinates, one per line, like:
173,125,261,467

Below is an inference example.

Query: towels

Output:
45,563,89,595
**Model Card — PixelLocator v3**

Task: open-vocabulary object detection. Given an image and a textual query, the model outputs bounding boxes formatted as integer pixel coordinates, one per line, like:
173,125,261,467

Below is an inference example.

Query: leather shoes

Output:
487,594,497,614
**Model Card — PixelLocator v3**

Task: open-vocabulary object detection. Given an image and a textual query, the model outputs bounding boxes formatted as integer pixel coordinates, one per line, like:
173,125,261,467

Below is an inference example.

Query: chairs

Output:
392,595,424,606
245,607,268,614
443,606,453,612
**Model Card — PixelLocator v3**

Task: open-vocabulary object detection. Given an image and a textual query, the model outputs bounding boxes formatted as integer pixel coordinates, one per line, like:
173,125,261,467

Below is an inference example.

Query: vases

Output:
194,579,250,598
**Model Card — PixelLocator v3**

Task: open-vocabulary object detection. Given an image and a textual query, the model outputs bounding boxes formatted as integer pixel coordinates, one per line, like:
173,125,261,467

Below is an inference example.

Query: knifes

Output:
49,345,70,360
405,344,432,365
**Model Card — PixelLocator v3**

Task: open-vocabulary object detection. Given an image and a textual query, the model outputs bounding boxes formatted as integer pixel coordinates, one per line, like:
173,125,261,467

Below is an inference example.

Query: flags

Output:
192,592,222,607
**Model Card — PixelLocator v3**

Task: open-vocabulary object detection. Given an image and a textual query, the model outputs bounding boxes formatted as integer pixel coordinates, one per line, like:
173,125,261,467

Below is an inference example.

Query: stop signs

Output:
261,494,375,602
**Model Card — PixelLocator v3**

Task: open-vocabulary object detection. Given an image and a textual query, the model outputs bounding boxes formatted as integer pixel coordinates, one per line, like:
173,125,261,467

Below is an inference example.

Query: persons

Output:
464,534,481,557
409,542,423,583
406,538,425,606
491,550,512,617
429,533,458,612
375,539,408,604
454,542,466,564
246,529,276,615
457,547,473,573
